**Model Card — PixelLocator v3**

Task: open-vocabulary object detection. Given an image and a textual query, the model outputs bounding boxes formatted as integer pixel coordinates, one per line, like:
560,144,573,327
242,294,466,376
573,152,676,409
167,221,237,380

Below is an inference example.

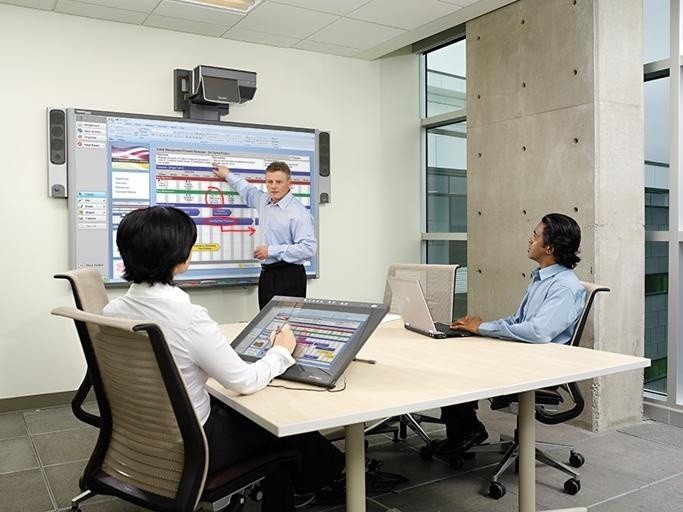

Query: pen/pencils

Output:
277,324,281,334
353,357,377,366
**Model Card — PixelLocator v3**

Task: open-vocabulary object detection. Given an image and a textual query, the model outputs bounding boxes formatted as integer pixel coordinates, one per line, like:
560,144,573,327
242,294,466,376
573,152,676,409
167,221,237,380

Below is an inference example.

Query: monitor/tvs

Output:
229,295,390,387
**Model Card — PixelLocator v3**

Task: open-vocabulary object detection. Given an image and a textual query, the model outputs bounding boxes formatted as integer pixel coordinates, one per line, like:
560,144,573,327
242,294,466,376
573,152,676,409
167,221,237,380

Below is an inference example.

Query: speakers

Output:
318,131,332,204
47,107,68,198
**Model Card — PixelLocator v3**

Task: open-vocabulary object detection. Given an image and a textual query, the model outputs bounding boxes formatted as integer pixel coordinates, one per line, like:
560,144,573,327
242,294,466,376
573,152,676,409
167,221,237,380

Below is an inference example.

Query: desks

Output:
204,322,651,511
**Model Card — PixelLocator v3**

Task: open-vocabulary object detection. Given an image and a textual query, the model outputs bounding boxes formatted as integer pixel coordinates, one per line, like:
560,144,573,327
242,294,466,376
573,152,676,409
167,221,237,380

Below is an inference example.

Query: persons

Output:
102,206,321,512
428,212,588,460
210,160,318,312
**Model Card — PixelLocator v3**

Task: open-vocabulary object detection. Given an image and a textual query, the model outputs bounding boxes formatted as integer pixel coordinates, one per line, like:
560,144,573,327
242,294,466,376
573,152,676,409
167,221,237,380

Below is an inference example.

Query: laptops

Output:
387,276,471,338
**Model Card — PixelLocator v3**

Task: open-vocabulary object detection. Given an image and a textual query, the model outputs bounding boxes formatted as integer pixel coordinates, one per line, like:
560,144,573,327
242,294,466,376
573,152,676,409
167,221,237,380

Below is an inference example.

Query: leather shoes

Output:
438,422,490,456
291,455,379,507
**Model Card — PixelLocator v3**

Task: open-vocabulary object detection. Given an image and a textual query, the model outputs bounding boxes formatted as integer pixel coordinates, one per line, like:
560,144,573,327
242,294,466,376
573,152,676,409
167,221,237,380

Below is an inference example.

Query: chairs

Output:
51,307,303,511
365,264,464,471
54,267,110,492
419,281,610,499
